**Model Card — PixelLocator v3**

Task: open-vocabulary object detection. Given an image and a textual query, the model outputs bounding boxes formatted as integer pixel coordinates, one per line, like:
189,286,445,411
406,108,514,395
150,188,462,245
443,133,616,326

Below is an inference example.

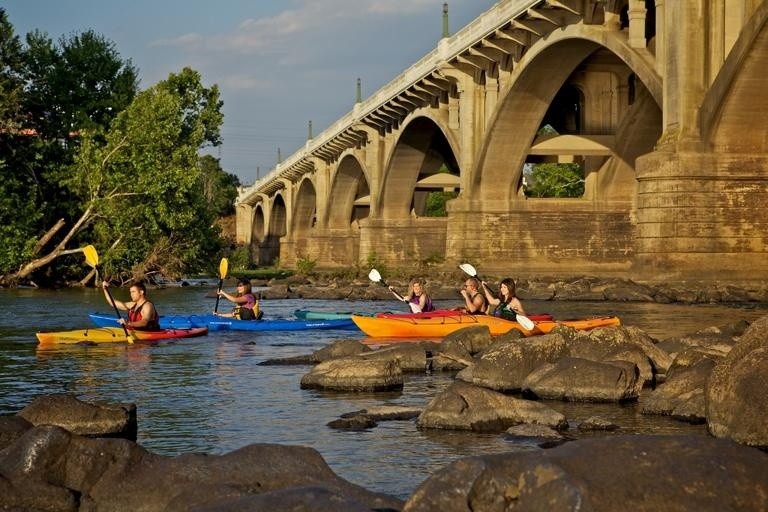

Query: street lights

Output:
307,120,313,140
256,166,260,180
442,1,450,39
276,147,282,164
356,77,362,103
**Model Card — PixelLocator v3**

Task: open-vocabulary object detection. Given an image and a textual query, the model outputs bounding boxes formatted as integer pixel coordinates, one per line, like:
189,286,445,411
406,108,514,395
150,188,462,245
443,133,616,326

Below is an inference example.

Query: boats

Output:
35,325,210,345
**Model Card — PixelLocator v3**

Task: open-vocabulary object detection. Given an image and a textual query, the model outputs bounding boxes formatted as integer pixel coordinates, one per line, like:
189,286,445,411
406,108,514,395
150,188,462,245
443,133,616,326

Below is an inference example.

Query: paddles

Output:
213,258,229,315
368,269,421,314
83,245,136,344
459,263,534,331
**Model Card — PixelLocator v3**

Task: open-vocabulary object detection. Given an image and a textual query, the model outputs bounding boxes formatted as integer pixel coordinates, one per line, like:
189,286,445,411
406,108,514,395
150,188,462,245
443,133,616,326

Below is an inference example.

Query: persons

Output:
388,278,434,315
212,279,260,320
102,279,161,332
450,278,486,314
480,277,525,321
